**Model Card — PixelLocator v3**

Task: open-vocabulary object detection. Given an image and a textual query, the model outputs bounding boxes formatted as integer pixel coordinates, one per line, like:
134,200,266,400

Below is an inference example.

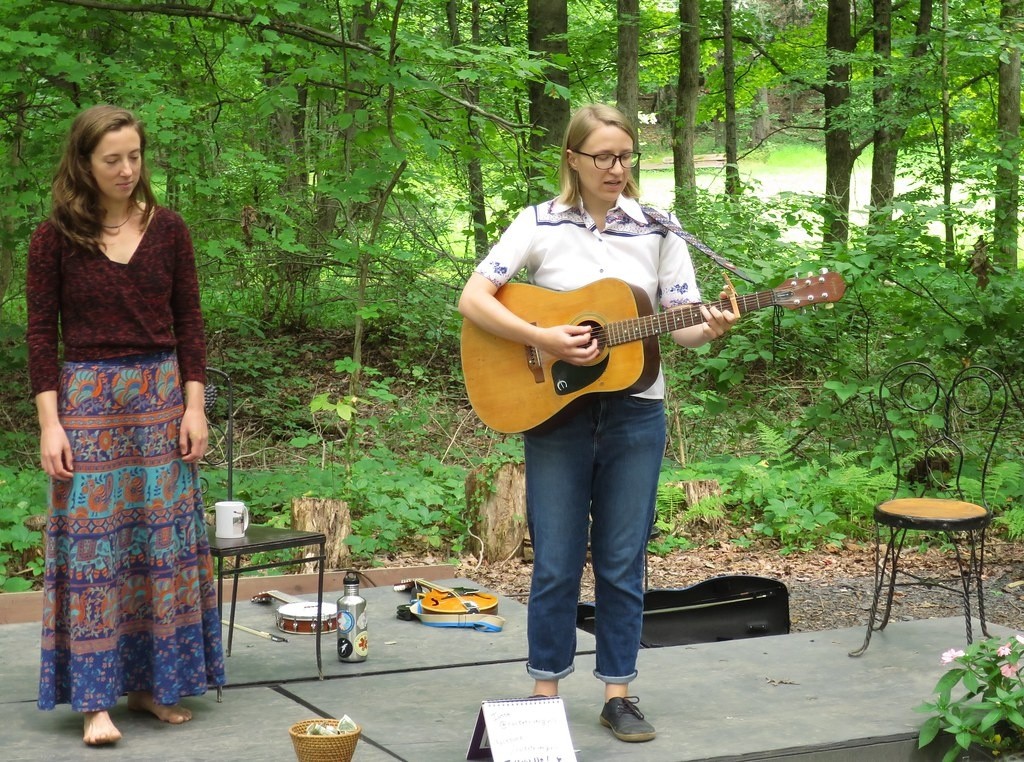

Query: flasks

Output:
337,570,368,662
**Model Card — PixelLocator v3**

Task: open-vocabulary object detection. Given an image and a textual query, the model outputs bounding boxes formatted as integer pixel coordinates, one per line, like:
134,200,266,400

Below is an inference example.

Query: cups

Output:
214,501,248,538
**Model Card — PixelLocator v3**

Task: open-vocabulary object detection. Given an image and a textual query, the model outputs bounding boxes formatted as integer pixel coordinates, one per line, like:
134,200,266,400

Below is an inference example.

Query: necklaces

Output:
105,211,132,227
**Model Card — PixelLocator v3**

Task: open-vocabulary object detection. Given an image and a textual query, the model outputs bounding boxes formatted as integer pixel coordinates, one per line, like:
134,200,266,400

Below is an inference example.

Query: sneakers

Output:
599,695,656,742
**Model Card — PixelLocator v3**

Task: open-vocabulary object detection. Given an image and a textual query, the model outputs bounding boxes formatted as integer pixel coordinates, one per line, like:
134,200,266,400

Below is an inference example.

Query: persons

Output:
27,105,225,746
459,104,740,742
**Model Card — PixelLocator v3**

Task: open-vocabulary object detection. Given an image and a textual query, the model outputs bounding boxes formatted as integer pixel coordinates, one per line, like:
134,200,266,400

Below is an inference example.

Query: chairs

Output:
182,367,327,700
850,360,1009,659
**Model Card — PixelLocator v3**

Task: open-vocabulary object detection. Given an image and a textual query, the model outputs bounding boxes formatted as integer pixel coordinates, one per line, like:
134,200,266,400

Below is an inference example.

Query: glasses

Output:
567,146,642,170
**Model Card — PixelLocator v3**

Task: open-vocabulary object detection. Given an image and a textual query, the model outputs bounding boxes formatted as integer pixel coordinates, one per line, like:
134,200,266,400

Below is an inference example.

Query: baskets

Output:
289,718,362,762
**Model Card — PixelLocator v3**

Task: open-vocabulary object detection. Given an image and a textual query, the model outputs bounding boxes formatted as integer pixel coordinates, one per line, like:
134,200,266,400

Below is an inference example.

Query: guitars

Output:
393,577,498,625
460,266,849,438
251,589,339,634
